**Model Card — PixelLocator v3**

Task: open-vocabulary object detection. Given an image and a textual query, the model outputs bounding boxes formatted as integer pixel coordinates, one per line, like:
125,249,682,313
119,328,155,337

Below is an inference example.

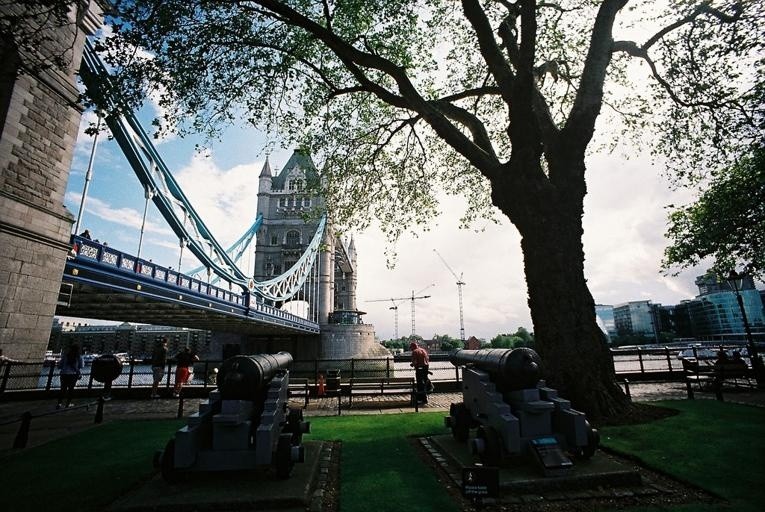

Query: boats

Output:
671,342,724,360
43,349,146,369
727,346,759,357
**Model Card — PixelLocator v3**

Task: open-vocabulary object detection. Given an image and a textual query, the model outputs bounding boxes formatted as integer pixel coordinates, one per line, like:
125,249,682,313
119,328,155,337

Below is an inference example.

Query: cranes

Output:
362,290,431,343
432,248,466,341
388,284,438,342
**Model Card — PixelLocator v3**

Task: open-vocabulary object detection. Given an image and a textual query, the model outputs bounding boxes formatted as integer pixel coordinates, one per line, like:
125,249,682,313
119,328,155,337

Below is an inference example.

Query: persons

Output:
710,350,729,392
731,352,748,370
171,347,199,398
410,342,434,391
150,337,168,398
56,344,85,409
79,230,90,240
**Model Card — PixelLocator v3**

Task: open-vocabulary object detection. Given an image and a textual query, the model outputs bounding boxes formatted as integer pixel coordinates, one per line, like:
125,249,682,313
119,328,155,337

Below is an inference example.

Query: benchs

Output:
288,378,312,410
680,354,755,399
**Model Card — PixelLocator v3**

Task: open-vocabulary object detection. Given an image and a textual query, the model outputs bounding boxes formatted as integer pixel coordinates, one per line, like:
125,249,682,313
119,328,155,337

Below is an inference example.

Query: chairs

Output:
345,375,417,405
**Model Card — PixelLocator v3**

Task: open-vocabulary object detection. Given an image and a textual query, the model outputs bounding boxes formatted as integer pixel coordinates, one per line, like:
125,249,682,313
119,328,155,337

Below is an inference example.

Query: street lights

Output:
724,268,764,390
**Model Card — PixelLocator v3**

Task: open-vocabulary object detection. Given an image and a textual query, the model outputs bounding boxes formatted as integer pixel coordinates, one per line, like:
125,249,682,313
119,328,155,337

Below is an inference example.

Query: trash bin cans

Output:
326,369,342,397
